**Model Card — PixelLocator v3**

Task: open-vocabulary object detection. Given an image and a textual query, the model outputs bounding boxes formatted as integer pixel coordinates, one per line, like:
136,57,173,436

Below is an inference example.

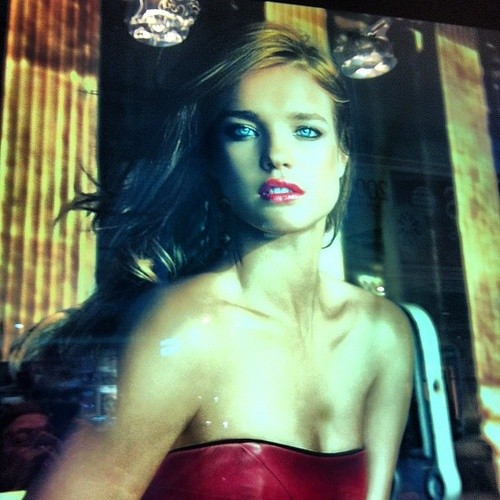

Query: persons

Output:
6,22,414,500
